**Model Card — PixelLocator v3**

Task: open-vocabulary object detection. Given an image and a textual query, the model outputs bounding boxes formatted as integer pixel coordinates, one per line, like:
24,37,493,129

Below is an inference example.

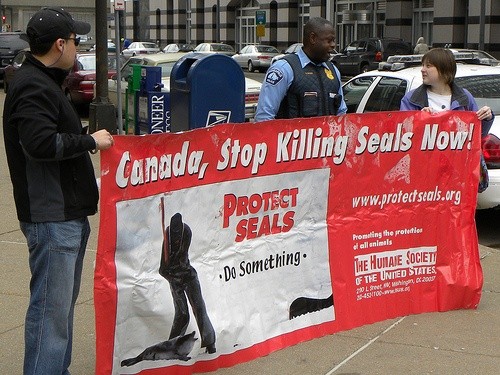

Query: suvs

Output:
0,32,30,81
330,36,415,78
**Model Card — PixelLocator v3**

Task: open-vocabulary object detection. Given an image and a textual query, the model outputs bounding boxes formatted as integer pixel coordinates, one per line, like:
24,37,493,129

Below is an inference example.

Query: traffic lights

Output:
2,15,6,25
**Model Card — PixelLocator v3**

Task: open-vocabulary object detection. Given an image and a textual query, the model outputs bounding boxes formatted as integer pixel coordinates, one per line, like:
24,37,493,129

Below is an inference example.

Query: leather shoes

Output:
205,343,216,354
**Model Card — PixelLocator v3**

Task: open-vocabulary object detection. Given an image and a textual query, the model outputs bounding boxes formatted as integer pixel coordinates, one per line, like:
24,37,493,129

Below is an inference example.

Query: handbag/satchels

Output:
478,154,489,193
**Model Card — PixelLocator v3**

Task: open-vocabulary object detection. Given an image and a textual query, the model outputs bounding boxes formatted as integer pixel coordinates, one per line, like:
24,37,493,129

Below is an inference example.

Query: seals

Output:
118,328,199,369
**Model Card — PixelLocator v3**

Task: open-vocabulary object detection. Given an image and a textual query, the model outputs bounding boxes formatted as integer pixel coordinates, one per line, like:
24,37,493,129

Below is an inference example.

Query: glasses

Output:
64,37,80,47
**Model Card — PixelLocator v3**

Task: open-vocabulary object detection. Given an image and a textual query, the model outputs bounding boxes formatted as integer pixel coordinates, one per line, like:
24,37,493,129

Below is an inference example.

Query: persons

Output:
254,17,348,122
400,49,495,193
3,7,114,375
159,212,216,354
414,37,429,54
121,38,131,50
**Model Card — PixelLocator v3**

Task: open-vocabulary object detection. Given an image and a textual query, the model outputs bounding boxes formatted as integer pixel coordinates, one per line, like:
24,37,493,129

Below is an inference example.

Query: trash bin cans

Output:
170,52,244,130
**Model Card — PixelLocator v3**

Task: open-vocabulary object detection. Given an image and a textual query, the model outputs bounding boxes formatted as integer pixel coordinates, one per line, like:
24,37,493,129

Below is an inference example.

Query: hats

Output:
27,8,91,46
170,212,182,233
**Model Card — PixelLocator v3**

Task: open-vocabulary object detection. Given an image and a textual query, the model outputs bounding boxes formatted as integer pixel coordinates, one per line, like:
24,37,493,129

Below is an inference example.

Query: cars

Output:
3,50,264,123
231,44,281,73
76,34,92,47
341,52,500,210
157,43,195,54
87,39,117,53
120,42,160,62
270,42,342,67
188,42,237,57
